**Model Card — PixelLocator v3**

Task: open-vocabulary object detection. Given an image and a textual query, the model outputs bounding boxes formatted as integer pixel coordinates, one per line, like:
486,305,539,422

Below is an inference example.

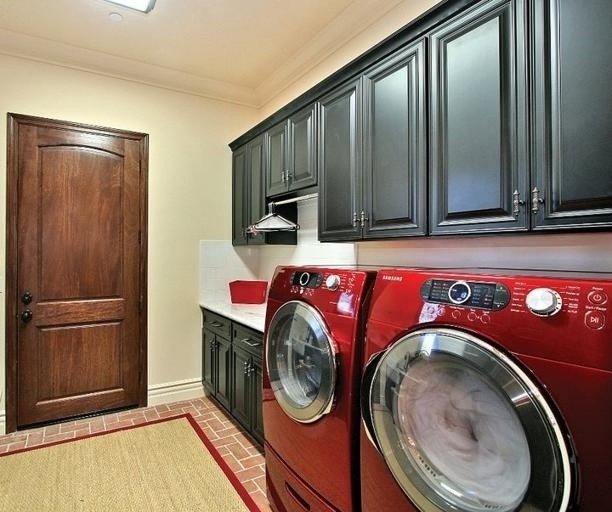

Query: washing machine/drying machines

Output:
261,266,376,512
360,270,611,512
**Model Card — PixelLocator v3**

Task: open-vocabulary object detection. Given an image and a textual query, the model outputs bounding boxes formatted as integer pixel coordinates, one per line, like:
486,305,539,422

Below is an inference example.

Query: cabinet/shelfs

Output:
234,323,264,356
203,328,230,412
319,34,426,241
265,103,320,196
202,309,232,362
233,344,265,445
232,135,263,244
428,0,612,234
